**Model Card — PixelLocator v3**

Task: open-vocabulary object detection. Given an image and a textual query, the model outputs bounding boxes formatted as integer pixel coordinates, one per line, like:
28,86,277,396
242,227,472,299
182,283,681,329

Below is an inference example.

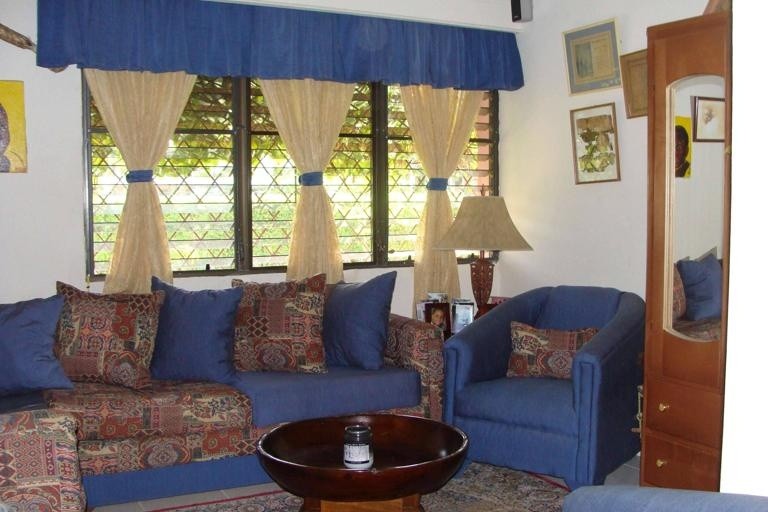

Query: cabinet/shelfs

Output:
638,10,730,493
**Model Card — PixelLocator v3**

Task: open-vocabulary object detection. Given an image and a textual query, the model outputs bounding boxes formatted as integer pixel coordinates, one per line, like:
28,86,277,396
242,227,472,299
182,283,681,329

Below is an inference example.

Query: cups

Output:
342,424,375,472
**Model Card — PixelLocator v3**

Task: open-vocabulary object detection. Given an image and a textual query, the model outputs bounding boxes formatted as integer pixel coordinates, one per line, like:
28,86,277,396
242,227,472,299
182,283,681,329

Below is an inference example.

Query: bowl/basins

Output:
257,412,469,501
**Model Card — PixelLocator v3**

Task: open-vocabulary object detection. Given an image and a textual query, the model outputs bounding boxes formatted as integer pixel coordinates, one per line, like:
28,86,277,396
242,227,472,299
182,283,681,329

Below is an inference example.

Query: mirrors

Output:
665,73,725,345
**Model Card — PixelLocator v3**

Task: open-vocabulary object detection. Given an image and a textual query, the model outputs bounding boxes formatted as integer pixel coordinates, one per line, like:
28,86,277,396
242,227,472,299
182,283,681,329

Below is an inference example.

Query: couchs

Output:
2,311,442,512
443,283,648,491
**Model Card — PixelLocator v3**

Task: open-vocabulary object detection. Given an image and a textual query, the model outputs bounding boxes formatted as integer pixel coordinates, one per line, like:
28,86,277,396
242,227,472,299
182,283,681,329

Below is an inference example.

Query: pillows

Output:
228,273,329,374
675,246,724,324
2,295,76,393
506,320,600,379
54,277,165,390
148,274,243,385
321,270,397,370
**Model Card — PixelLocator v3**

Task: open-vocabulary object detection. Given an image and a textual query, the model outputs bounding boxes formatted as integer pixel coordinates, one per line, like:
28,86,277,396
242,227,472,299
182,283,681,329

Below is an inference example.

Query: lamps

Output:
429,196,534,320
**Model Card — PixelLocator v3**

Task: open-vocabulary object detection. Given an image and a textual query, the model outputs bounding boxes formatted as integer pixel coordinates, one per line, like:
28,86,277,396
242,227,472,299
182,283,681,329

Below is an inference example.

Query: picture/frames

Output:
0,81,28,172
568,102,622,185
451,300,474,335
422,302,453,342
561,17,625,97
620,49,649,119
692,95,726,143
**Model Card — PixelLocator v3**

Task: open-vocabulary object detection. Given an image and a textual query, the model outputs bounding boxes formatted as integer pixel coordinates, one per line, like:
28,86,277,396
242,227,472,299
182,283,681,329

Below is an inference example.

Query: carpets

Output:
150,459,571,511
